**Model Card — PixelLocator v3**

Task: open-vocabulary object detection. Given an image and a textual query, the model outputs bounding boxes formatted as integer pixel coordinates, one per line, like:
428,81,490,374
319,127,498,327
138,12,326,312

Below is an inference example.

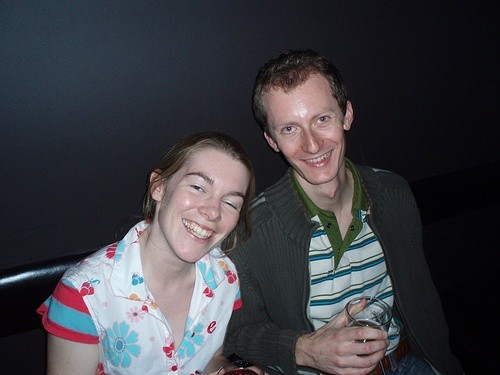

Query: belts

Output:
366,341,412,375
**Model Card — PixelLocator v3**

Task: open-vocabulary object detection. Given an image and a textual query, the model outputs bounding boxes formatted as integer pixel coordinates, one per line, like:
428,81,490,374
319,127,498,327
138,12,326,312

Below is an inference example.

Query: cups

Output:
345,296,393,342
217,360,265,375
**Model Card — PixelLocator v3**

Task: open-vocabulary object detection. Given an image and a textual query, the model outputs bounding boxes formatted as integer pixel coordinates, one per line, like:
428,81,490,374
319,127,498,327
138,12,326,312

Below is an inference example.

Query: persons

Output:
221,49,466,375
37,131,269,375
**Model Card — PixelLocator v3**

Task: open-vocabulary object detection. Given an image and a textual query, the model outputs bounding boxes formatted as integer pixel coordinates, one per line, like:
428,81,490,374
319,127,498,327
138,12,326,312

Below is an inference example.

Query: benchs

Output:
0,249,99,375
